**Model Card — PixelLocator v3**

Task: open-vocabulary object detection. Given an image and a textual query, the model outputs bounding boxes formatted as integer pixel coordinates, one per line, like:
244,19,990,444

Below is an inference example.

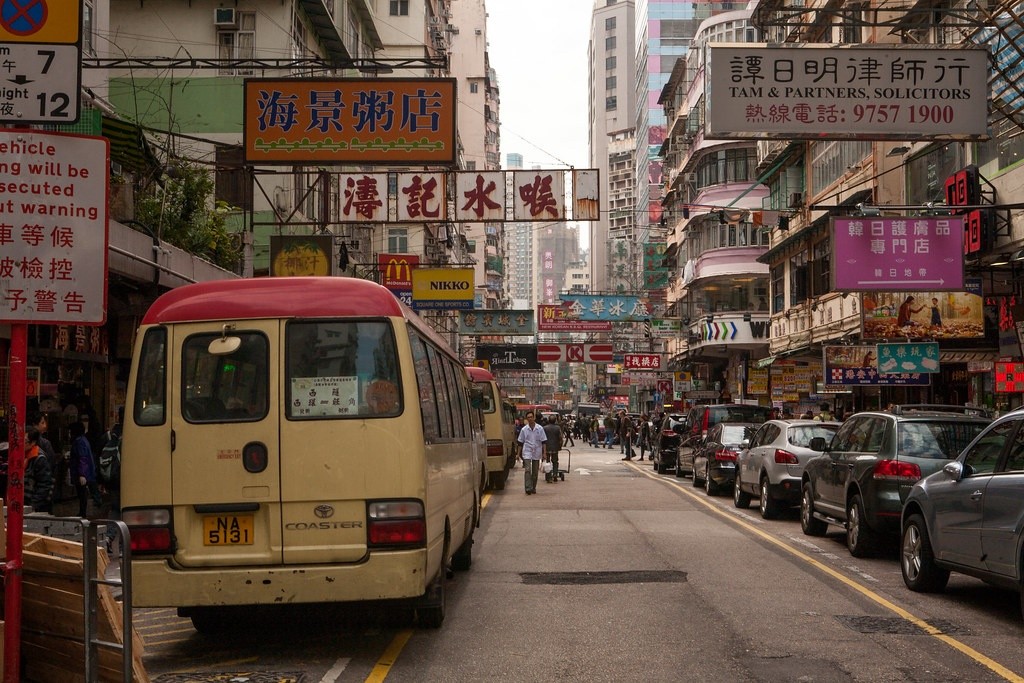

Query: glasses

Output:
80,418,89,422
890,407,893,408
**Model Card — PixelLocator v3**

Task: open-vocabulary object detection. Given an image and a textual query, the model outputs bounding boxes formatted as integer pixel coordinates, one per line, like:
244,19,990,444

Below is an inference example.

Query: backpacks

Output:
100,430,121,483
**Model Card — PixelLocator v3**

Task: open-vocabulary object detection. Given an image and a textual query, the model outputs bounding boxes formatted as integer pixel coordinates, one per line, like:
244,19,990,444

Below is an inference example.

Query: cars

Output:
691,422,764,497
797,403,993,559
733,418,845,519
651,413,688,474
595,412,654,442
673,404,772,478
899,409,1024,595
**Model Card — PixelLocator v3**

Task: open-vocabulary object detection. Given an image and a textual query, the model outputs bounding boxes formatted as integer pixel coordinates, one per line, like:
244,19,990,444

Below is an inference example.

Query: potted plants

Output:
763,139,777,160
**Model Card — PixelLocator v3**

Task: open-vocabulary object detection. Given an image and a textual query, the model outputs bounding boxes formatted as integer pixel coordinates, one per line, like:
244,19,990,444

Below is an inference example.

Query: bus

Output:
118,275,486,630
465,367,553,490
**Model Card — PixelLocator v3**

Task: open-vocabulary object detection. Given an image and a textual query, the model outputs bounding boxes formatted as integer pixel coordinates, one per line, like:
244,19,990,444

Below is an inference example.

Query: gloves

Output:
79,477,87,486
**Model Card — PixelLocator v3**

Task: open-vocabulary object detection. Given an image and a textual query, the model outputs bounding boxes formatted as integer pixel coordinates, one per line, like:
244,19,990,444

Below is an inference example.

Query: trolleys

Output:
553,449,571,482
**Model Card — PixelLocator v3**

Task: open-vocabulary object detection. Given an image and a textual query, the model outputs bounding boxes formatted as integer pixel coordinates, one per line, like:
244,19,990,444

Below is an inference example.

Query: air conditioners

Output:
669,168,678,183
663,212,669,219
789,191,802,207
429,74,437,78
678,183,685,193
487,227,496,234
663,100,671,116
784,17,798,42
667,271,676,283
757,140,765,166
350,240,364,253
684,172,697,184
966,0,991,30
430,9,455,51
673,192,680,201
475,29,481,34
213,8,239,28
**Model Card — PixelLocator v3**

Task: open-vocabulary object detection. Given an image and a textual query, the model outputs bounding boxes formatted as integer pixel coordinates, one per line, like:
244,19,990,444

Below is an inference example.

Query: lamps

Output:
885,146,911,158
811,301,818,312
840,291,850,300
785,308,799,320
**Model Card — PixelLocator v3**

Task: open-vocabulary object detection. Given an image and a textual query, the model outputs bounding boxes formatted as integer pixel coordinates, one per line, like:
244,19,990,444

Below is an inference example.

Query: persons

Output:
25,407,126,518
772,402,855,423
886,402,896,413
898,296,926,327
925,298,941,326
515,411,666,495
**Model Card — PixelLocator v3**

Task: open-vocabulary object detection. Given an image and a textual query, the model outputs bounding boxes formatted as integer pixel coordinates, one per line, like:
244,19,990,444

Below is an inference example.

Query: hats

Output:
0,442,9,450
820,402,829,409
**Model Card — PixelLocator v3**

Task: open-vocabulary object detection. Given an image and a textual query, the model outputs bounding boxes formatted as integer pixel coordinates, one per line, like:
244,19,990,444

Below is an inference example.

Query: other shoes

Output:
570,445,575,447
589,442,591,446
562,445,566,447
526,491,536,495
547,476,558,483
579,438,582,439
603,444,605,448
620,450,624,454
637,459,644,461
622,458,630,461
595,446,600,448
76,513,86,518
636,445,639,448
523,466,525,468
608,447,614,449
632,452,636,457
